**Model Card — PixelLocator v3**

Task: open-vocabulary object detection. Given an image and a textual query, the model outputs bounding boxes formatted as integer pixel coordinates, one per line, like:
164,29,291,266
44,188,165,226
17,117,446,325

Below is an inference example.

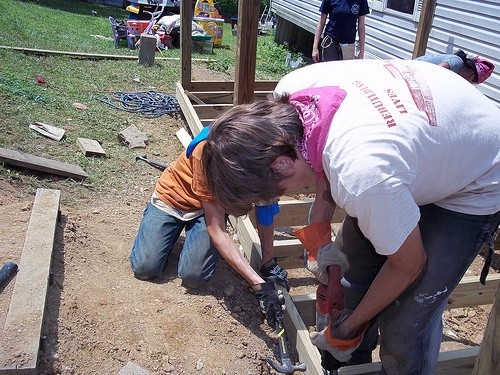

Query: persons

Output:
412,50,495,84
129,124,292,330
312,0,370,63
201,58,500,375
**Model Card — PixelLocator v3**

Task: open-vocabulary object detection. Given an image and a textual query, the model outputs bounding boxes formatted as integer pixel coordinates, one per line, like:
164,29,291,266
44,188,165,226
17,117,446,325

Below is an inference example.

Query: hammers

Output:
135,153,166,172
264,301,306,374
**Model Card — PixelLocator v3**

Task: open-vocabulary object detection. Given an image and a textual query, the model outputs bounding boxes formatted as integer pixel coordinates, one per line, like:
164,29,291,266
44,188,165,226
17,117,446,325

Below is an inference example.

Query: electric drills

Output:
316,265,350,375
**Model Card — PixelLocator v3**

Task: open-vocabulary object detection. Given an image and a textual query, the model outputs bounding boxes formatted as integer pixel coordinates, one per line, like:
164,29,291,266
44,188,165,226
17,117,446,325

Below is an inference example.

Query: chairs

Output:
109,17,136,49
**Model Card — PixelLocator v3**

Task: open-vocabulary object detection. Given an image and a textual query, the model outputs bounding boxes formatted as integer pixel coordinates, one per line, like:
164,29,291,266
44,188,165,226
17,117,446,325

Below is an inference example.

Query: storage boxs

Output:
127,20,150,33
